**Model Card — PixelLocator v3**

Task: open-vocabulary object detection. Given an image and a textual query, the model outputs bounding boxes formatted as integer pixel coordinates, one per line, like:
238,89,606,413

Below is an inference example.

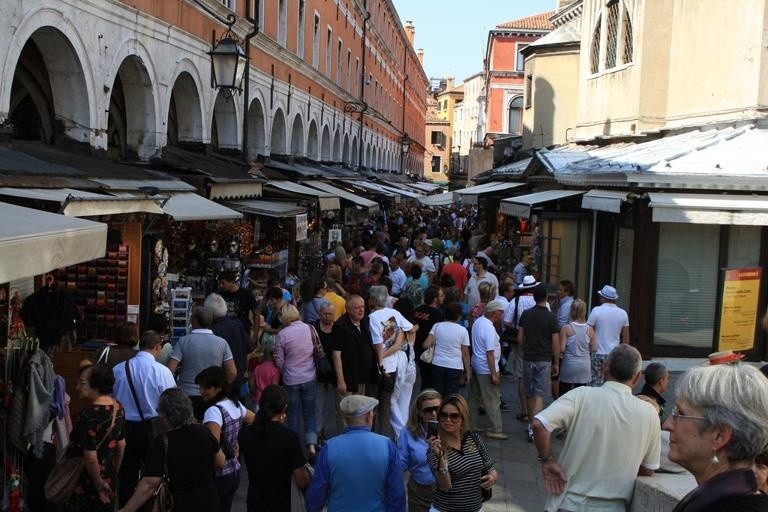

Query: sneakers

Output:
487,431,511,440
528,424,537,443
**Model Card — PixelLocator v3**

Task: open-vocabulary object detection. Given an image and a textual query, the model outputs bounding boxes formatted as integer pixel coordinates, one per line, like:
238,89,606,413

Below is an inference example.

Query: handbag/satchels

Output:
307,323,337,384
502,295,519,343
43,397,116,505
470,432,492,499
133,431,174,512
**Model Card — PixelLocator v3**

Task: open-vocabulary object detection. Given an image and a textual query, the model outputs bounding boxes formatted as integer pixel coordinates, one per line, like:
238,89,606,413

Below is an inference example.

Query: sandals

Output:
516,413,531,421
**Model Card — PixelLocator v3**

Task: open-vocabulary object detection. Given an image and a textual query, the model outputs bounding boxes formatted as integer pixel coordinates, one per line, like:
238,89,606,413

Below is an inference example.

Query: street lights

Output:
400,135,409,175
203,12,258,163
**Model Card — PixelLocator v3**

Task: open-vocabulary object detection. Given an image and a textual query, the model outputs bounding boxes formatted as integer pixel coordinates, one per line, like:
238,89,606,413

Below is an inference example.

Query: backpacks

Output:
504,296,519,343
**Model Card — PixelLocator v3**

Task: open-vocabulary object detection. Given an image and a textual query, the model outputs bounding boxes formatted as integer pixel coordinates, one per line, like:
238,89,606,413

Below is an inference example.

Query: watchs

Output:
537,454,555,462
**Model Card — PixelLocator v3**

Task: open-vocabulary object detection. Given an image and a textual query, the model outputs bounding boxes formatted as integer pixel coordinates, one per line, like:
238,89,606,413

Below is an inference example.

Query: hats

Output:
598,286,618,299
519,275,541,288
485,299,510,313
340,395,379,418
701,350,746,365
423,240,434,248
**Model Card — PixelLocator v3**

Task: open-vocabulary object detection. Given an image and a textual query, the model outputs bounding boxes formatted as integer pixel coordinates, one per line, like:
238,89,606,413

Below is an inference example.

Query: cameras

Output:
426,421,439,441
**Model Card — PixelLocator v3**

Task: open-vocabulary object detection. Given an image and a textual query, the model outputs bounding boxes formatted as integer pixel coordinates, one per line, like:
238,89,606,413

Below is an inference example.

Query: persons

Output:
586,285,630,387
398,255,407,267
387,255,406,296
472,203,478,219
426,394,497,512
255,348,280,412
444,247,456,265
479,203,487,229
468,282,502,333
360,263,383,300
559,299,597,397
217,270,260,379
504,275,551,422
407,246,436,289
500,271,515,284
392,237,415,258
436,274,461,302
321,277,346,321
488,266,499,275
333,245,348,270
422,240,439,275
531,343,661,512
360,239,382,269
661,364,768,511
400,264,424,307
412,285,444,373
312,302,337,448
633,363,669,425
80,322,139,366
238,384,310,511
749,441,768,496
500,281,514,299
118,387,226,512
422,303,471,397
67,363,126,512
113,330,177,495
367,199,474,245
464,257,499,306
266,275,298,323
518,287,559,437
194,365,257,511
262,288,291,349
274,304,324,467
167,306,237,422
513,257,529,285
446,235,461,249
476,246,495,268
556,280,574,329
430,232,443,249
367,286,419,440
398,389,442,511
471,300,512,422
350,255,369,291
326,264,343,295
306,394,406,512
705,350,746,365
442,288,469,329
441,251,467,297
204,293,248,400
331,295,378,434
299,277,330,323
383,298,416,445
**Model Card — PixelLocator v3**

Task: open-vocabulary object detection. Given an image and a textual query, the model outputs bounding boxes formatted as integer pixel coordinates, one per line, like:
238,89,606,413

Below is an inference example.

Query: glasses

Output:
322,286,329,291
672,409,712,426
420,407,440,415
474,262,481,265
438,412,463,421
